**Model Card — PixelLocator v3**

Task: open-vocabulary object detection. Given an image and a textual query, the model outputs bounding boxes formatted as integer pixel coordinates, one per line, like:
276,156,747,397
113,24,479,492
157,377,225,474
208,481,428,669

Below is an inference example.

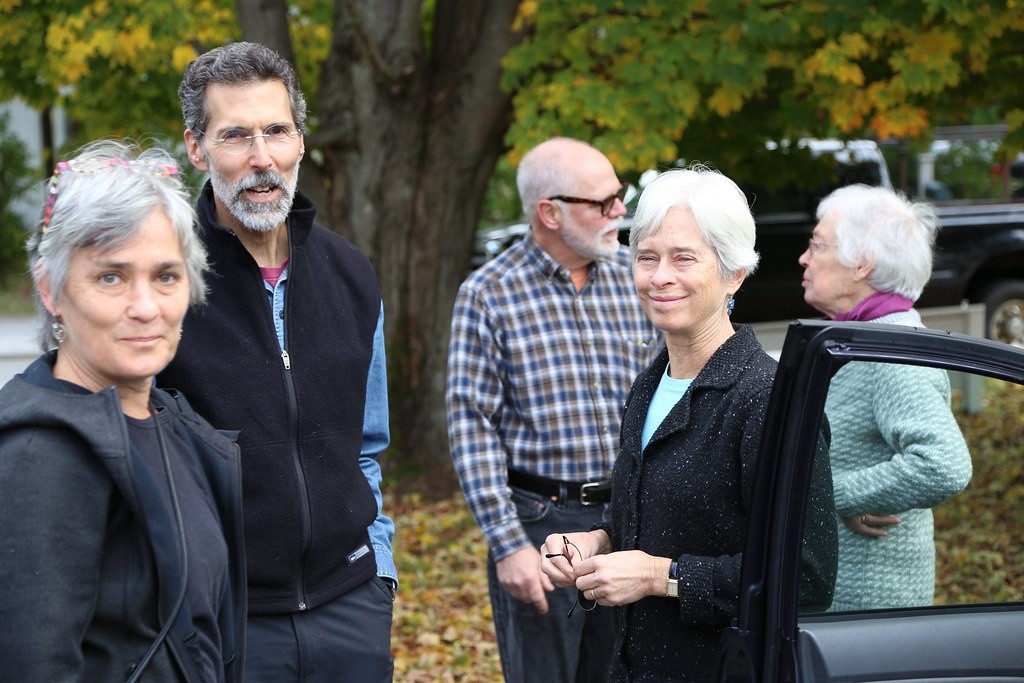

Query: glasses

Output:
548,188,627,216
808,239,837,255
195,122,301,154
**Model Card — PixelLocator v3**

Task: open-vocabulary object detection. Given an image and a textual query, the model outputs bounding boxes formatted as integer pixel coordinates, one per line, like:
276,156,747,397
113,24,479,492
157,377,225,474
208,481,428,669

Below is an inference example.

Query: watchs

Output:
666,558,679,598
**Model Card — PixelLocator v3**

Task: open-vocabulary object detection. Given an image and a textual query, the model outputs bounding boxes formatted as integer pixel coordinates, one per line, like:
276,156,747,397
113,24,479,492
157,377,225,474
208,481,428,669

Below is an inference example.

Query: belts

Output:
507,468,612,506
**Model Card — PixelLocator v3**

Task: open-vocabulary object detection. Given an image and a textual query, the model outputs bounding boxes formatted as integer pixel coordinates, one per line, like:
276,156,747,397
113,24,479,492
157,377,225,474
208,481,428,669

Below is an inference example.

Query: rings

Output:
591,589,598,599
862,514,866,524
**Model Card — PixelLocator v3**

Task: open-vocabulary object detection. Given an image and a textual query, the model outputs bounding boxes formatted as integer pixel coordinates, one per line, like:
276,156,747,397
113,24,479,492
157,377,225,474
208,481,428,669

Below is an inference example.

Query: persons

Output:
540,168,839,683
799,183,973,612
156,41,399,683
0,137,244,683
445,136,667,683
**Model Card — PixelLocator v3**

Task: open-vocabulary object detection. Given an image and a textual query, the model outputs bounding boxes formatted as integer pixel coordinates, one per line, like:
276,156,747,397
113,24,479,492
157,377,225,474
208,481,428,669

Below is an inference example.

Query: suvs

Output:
470,122,1024,351
